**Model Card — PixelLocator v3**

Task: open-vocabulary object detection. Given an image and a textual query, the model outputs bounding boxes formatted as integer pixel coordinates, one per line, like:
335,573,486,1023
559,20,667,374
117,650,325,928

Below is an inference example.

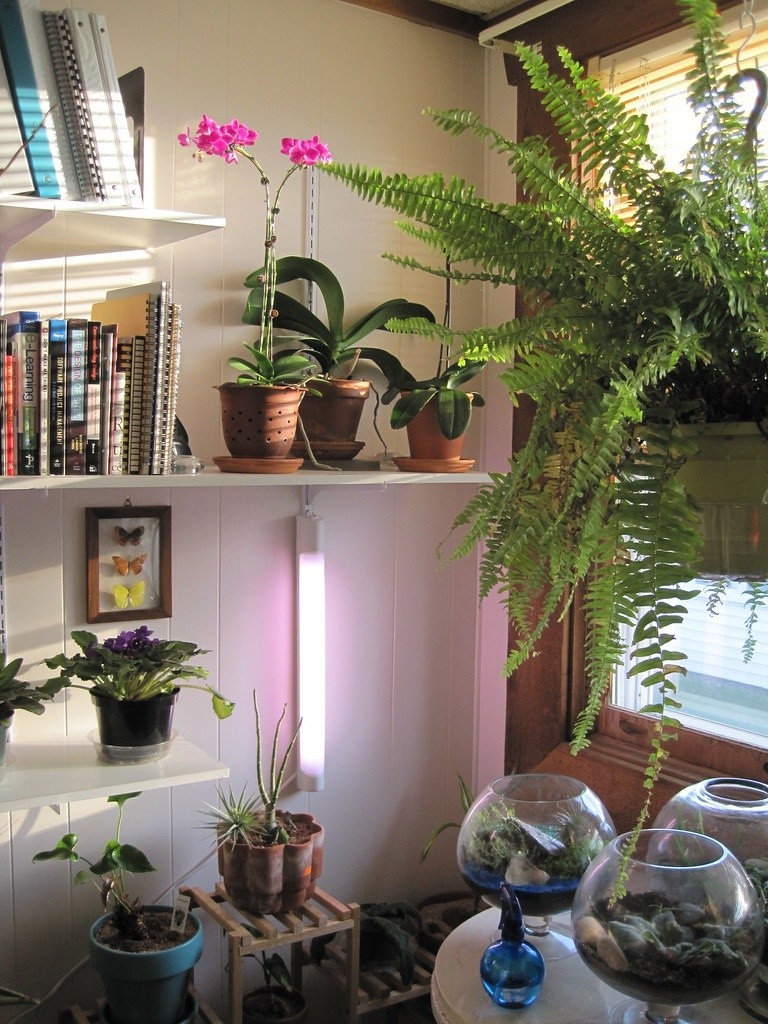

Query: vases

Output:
211,380,309,474
89,681,181,766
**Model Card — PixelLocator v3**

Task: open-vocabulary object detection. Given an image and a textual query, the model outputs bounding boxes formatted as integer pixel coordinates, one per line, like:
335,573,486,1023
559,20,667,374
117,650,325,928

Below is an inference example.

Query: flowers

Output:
31,625,236,719
177,113,336,399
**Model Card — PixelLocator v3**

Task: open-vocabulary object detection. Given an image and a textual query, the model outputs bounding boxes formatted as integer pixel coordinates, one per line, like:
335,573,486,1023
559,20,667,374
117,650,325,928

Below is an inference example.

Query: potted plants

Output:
1,652,55,767
241,256,436,460
311,0,768,908
189,689,325,914
221,920,309,1024
381,353,485,473
309,899,423,989
421,770,484,936
31,790,204,1024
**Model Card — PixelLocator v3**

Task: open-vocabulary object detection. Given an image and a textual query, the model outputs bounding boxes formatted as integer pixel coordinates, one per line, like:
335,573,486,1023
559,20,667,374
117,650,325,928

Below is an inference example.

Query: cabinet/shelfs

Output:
0,194,508,848
173,877,452,1024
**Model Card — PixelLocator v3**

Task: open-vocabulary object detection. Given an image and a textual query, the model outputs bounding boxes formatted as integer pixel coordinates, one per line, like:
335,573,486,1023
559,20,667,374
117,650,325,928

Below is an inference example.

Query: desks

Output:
431,906,768,1024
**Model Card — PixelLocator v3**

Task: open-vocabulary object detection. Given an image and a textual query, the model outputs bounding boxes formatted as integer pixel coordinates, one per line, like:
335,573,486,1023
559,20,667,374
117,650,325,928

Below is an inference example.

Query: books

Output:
1,0,147,209
0,279,183,476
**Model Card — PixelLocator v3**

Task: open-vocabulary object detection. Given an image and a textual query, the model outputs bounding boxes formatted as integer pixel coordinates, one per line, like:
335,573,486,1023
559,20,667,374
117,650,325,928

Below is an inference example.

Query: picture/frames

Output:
85,506,173,624
